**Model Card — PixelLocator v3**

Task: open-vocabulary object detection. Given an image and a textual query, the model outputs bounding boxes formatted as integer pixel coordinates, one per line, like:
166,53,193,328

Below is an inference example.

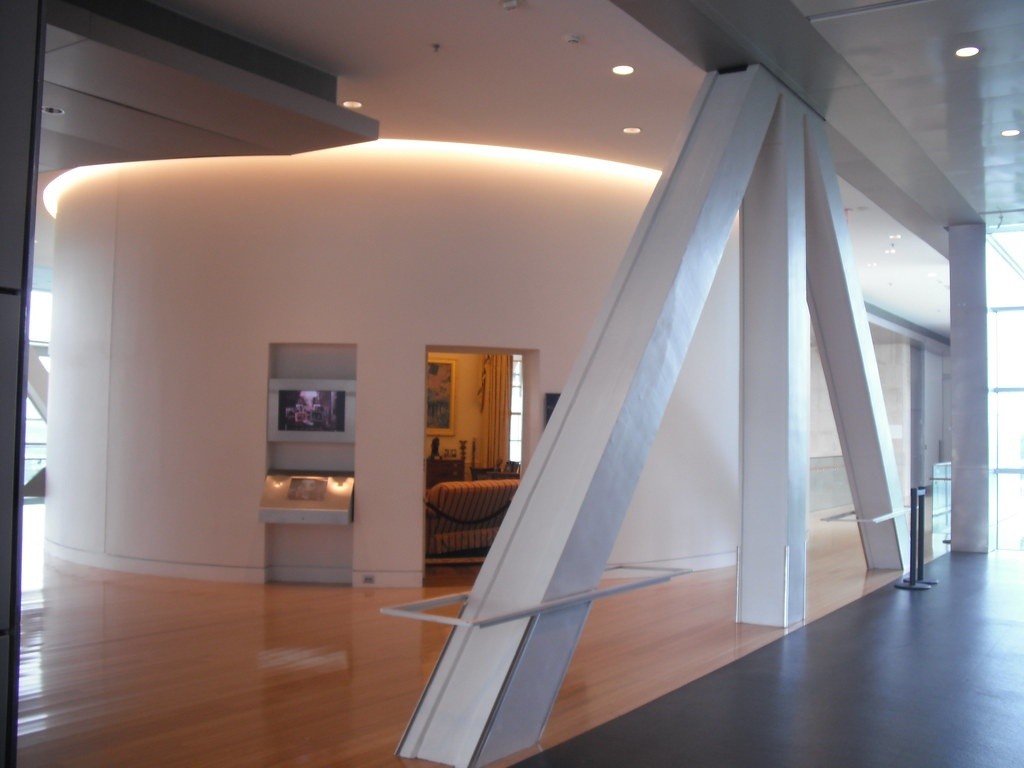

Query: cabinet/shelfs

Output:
425,457,464,489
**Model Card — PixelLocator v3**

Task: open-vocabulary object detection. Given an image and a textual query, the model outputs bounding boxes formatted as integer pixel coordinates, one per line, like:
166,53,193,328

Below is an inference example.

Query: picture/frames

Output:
424,358,457,438
266,378,354,444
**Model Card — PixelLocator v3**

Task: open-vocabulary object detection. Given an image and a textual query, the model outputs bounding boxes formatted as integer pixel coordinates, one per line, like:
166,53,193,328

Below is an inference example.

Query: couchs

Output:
425,477,520,557
